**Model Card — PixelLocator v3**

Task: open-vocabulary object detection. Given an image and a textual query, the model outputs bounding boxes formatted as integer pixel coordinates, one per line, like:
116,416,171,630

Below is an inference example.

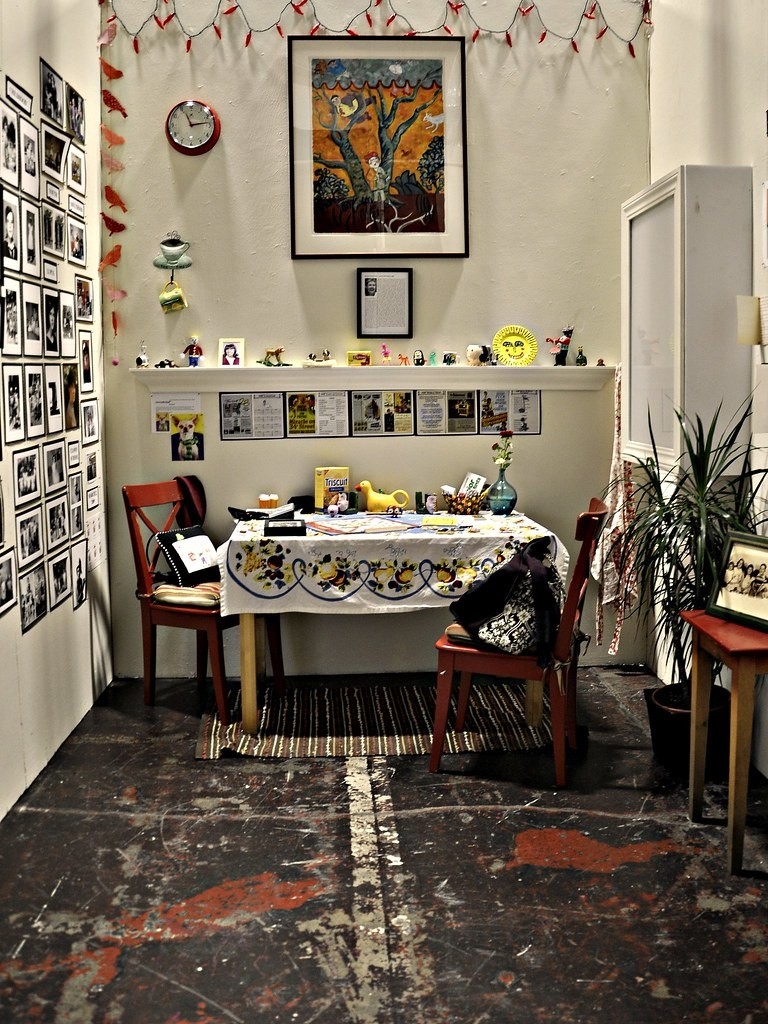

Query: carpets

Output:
196,683,567,759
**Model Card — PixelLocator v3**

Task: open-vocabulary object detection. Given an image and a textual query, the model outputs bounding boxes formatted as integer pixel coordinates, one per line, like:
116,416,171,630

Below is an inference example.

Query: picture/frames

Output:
218,338,244,368
357,268,413,339
287,35,469,258
705,530,768,635
0,56,99,636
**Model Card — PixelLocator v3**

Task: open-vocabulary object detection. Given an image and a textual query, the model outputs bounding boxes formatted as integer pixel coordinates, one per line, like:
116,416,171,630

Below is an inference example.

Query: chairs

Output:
428,497,608,786
121,480,286,725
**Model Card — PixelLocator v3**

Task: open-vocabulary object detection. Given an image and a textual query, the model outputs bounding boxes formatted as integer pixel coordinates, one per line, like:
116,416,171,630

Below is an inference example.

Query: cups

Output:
159,239,190,264
159,281,188,314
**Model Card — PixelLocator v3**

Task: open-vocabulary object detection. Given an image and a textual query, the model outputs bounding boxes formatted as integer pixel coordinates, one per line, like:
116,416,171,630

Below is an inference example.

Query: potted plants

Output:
595,381,768,773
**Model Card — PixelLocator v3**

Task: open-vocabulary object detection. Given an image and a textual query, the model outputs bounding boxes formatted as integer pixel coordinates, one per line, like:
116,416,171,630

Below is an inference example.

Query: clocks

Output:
165,99,220,156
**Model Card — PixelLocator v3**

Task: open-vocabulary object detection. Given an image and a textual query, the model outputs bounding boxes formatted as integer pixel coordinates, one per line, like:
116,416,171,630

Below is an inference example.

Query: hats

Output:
172,475,207,529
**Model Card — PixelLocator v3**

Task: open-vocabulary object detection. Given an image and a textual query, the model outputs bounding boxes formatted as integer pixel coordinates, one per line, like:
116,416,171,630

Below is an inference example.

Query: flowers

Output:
492,431,514,501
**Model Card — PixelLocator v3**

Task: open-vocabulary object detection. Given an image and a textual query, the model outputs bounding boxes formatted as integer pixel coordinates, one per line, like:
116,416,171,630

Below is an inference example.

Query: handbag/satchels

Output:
146,524,223,589
450,532,566,669
159,281,188,315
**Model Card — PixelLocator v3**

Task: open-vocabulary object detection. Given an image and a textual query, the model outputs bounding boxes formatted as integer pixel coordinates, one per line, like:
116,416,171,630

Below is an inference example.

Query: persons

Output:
0,116,92,624
181,335,204,368
723,559,768,597
223,344,239,365
365,278,376,296
43,72,78,129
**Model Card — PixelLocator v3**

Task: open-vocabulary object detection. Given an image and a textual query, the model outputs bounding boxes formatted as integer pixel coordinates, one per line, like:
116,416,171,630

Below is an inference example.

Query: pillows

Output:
155,525,221,588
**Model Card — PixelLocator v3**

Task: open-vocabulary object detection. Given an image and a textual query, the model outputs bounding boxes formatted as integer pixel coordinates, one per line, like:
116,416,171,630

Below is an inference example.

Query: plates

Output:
492,324,538,365
152,254,193,269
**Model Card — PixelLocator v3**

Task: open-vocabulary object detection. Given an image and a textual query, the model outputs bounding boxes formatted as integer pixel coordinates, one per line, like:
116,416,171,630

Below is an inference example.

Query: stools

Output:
680,610,768,876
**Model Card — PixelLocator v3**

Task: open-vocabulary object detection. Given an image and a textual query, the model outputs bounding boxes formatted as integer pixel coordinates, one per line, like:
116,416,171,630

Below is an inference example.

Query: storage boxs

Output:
315,467,349,515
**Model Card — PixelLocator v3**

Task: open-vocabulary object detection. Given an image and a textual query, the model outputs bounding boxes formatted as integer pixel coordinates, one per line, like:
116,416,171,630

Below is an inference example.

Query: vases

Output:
487,468,517,515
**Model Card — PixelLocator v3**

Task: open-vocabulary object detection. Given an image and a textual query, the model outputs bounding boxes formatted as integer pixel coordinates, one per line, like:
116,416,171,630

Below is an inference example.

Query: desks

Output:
218,509,569,733
